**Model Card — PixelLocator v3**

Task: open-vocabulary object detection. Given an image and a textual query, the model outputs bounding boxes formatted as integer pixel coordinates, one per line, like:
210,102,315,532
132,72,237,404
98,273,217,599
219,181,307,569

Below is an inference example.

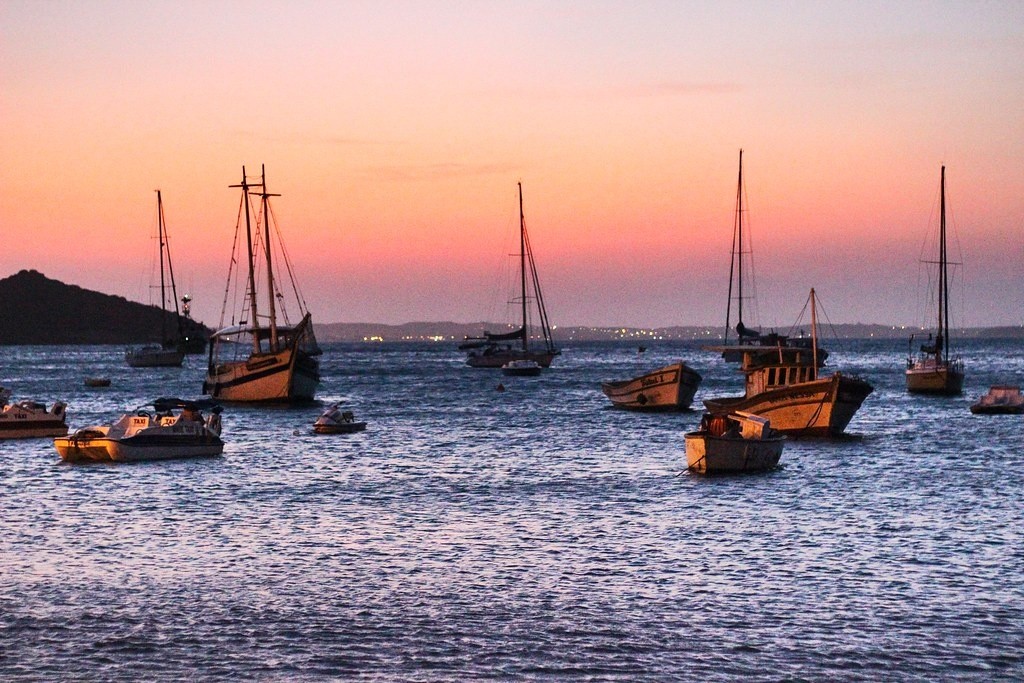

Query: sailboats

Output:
140,187,210,355
457,180,564,369
697,144,830,373
903,163,968,396
703,285,875,439
201,163,323,408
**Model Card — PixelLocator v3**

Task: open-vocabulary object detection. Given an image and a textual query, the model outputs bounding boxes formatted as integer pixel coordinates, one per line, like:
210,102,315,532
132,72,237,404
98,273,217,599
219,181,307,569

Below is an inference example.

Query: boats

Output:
123,341,187,367
682,409,789,476
53,401,225,463
312,405,368,435
969,383,1024,415
501,360,544,376
599,358,704,414
0,386,70,439
